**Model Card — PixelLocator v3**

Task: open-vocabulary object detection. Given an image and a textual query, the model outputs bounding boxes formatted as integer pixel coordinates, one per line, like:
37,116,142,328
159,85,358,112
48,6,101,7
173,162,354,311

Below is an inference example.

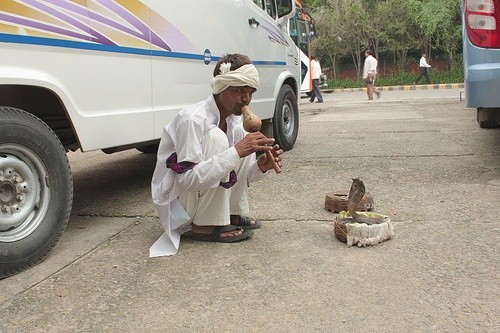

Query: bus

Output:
1,0,319,281
458,0,500,129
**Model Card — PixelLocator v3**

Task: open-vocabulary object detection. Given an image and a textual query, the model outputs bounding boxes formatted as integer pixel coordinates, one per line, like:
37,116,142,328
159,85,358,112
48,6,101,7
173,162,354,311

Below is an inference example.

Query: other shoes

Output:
368,98,373,100
315,101,323,103
309,100,313,102
376,92,380,98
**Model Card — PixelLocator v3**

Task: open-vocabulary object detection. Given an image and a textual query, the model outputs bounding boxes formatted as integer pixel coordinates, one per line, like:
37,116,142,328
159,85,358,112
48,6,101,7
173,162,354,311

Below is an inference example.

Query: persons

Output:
147,54,284,258
413,53,432,84
362,50,380,100
309,56,324,103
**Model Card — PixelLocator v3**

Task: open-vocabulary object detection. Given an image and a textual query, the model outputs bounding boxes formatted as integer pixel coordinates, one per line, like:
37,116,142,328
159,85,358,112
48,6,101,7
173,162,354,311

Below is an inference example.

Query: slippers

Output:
192,224,254,243
230,215,262,230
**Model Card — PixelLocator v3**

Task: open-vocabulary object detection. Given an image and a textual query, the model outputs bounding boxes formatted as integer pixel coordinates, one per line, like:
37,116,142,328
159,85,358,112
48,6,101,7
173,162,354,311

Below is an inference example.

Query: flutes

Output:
241,105,282,174
